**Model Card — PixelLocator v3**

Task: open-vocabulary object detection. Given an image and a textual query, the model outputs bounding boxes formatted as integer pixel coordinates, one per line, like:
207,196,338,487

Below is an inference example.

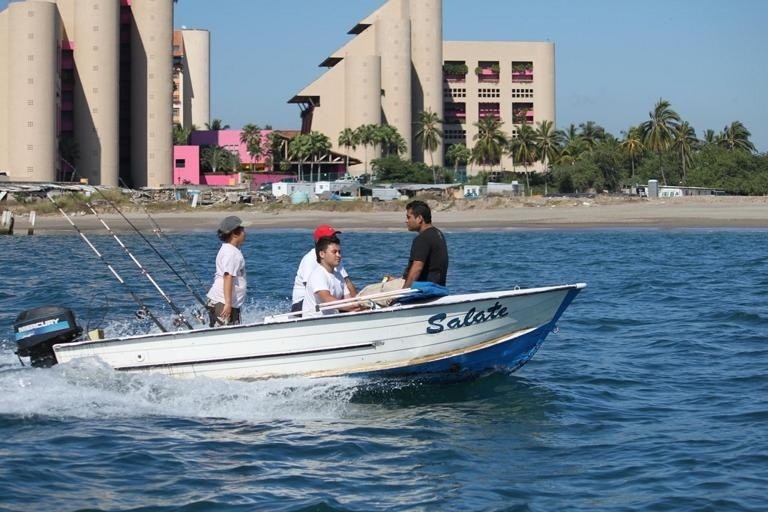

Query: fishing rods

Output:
39,157,220,332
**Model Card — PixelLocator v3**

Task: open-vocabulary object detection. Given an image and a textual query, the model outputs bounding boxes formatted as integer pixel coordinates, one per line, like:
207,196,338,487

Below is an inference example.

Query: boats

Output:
12,276,588,392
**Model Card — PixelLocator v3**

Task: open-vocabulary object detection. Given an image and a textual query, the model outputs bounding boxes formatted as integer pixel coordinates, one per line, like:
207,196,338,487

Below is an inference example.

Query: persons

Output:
203,216,252,326
290,224,357,318
402,199,447,289
299,235,368,319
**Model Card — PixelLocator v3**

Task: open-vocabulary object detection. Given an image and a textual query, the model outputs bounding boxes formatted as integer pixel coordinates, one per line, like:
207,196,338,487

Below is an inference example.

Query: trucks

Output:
371,188,402,202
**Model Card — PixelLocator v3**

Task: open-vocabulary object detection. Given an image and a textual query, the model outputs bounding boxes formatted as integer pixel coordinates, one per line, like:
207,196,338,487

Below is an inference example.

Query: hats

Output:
220,216,251,234
314,225,342,241
